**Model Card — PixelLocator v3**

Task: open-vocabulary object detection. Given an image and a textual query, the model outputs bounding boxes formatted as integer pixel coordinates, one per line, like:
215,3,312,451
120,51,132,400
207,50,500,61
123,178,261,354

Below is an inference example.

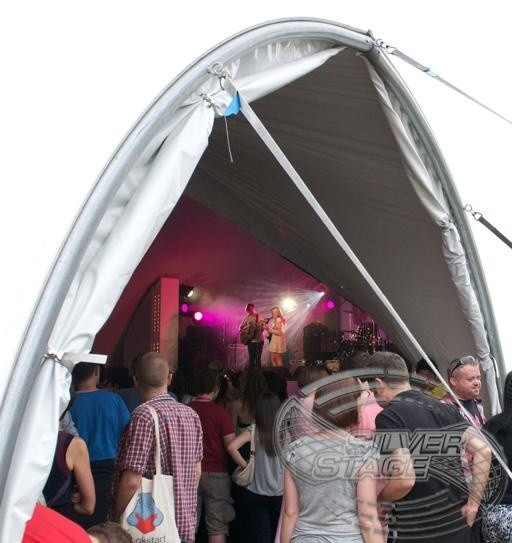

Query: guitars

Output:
240,318,273,345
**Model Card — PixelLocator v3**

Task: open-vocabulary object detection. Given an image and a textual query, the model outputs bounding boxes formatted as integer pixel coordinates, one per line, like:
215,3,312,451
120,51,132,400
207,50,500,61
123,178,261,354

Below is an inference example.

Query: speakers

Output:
302,327,328,359
189,324,218,360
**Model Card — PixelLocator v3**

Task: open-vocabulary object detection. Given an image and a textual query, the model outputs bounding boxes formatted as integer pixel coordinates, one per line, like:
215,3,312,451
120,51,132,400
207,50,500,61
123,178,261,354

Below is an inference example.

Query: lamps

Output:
179,284,206,325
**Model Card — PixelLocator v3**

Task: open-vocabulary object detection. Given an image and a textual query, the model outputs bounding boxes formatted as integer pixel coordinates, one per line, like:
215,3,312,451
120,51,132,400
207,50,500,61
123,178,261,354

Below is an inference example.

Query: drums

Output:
337,328,386,355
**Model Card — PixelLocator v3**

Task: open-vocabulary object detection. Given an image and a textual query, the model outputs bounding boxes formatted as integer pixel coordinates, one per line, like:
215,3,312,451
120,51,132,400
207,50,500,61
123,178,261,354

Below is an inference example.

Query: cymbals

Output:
344,310,355,313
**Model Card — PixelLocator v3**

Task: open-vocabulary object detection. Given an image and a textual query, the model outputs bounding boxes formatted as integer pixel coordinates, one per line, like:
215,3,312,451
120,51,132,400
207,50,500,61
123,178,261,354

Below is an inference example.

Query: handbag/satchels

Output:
119,475,182,543
230,424,257,487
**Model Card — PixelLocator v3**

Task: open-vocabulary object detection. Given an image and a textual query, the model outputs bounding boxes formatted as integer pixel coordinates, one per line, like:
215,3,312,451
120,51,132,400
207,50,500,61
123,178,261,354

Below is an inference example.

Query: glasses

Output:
450,355,475,376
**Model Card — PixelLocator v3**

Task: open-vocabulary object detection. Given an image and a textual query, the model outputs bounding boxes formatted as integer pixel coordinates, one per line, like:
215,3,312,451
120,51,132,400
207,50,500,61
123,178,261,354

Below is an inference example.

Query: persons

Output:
262,307,286,367
239,304,265,368
